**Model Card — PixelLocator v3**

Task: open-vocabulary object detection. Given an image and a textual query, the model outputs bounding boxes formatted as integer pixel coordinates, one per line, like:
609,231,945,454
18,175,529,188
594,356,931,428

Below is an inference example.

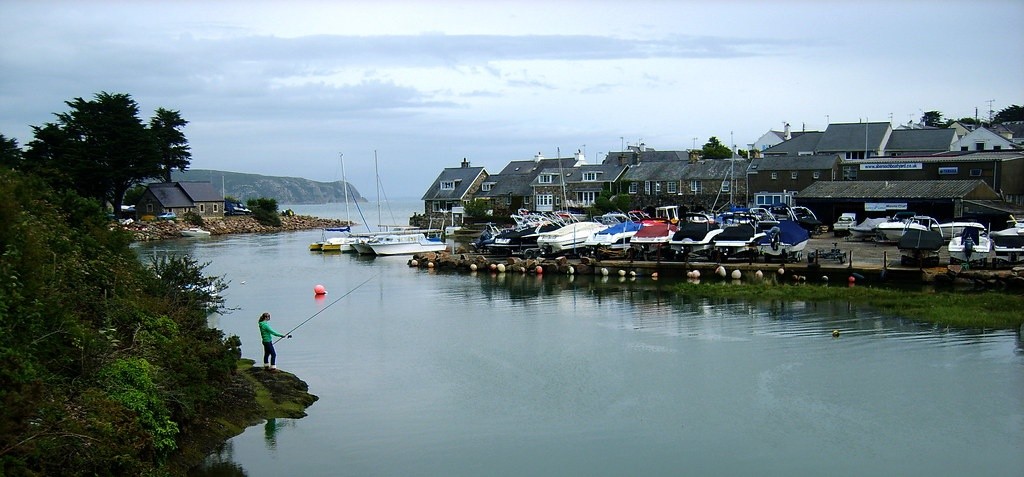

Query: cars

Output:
223,205,253,216
157,212,177,221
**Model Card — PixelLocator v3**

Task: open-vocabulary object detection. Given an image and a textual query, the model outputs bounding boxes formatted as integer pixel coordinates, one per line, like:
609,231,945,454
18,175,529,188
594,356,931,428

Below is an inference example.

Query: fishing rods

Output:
269,273,383,346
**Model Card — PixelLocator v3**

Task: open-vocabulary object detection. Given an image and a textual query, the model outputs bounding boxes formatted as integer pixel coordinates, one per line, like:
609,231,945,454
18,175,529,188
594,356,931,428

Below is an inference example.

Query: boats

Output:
849,211,1024,268
464,145,828,263
178,228,212,238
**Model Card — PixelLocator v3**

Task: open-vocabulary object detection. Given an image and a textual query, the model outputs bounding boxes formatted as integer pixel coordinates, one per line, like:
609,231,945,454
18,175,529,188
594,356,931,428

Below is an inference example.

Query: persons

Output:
259,313,285,373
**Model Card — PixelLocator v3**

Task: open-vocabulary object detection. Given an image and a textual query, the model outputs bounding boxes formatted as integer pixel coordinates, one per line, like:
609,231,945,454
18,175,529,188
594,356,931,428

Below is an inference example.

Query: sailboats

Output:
308,149,450,257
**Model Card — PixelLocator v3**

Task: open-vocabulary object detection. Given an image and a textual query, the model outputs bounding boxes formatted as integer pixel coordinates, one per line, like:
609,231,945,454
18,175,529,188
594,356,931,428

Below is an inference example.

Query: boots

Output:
264,363,280,373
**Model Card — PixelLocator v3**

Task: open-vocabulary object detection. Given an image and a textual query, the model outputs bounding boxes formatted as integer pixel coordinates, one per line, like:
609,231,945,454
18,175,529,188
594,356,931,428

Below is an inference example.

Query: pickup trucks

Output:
832,215,858,236
